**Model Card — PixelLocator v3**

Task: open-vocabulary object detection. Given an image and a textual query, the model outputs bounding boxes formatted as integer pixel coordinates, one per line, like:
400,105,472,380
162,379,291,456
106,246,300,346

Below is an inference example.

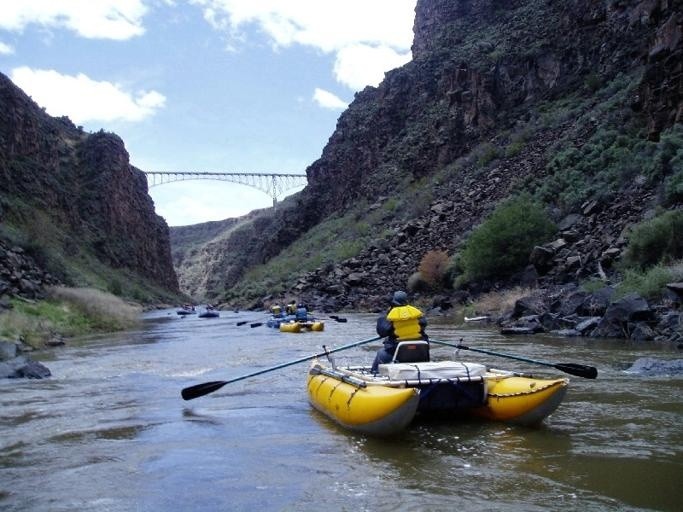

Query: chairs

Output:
391,340,429,363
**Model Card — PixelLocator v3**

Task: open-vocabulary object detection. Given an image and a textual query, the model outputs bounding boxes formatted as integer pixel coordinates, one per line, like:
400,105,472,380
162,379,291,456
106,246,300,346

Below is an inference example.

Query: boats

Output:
177,309,196,315
199,309,219,318
267,314,315,328
305,355,569,438
278,320,324,334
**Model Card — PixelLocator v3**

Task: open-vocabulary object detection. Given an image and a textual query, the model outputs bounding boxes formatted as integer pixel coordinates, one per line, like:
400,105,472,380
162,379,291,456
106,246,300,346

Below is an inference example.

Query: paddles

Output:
309,316,339,319
251,318,295,328
181,336,384,401
427,339,598,380
237,315,275,326
313,319,348,322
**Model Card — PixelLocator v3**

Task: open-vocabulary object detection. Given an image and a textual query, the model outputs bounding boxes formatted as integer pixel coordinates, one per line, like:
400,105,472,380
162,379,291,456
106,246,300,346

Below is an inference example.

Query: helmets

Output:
298,304,303,308
391,291,408,306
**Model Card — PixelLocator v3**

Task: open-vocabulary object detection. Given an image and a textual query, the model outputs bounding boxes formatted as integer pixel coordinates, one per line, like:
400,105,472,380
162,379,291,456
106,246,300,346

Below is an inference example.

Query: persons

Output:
286,300,296,314
181,303,189,311
294,303,307,320
205,304,213,311
189,305,196,311
372,290,429,373
271,302,282,314
